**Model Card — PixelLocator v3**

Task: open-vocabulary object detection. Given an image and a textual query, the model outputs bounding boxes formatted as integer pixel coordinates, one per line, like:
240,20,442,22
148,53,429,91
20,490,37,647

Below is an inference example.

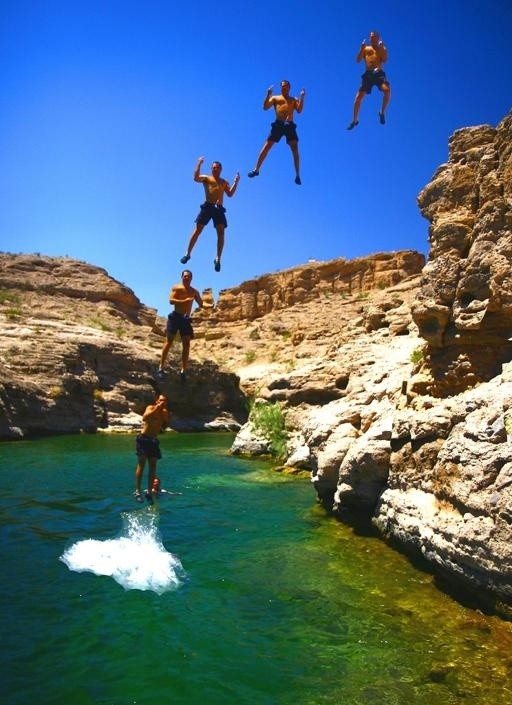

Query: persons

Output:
131,393,171,505
148,477,161,495
180,157,241,273
346,30,391,131
246,79,306,185
157,270,214,382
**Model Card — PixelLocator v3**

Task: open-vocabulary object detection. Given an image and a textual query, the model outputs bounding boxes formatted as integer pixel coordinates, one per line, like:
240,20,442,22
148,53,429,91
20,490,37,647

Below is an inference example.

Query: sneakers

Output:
295,177,301,186
248,169,258,178
378,111,385,124
145,491,154,506
214,258,220,272
159,368,166,378
180,370,187,379
133,493,144,503
347,120,358,129
180,256,190,263
